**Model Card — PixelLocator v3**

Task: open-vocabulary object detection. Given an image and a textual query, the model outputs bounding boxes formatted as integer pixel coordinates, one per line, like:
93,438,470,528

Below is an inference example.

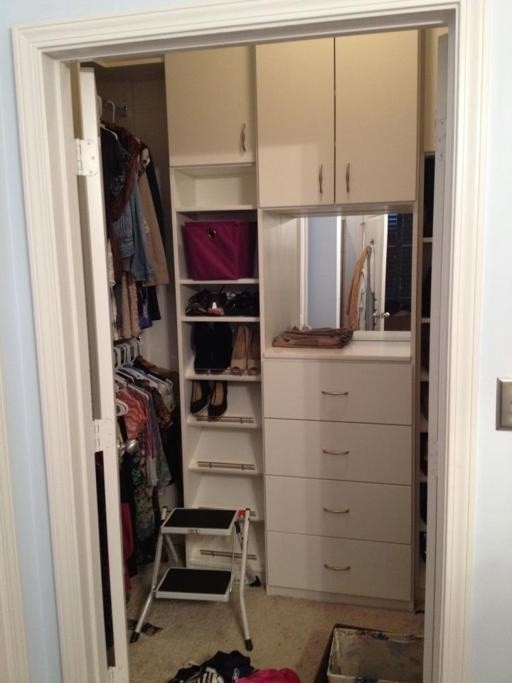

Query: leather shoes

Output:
193,323,213,372
212,322,231,373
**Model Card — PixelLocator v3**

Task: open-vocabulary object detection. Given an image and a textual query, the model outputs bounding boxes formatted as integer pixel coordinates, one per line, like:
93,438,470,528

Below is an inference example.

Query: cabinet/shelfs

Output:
165,31,422,613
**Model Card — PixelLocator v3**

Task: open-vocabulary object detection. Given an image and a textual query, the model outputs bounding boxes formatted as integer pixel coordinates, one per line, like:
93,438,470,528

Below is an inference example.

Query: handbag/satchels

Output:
182,219,255,281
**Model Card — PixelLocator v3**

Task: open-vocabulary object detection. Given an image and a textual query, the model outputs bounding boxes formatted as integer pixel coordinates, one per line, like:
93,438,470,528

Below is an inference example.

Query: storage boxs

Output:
313,623,424,683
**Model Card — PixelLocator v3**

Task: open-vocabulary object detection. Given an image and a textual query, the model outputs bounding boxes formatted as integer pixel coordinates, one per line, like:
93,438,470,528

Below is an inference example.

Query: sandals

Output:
249,328,262,375
232,326,248,373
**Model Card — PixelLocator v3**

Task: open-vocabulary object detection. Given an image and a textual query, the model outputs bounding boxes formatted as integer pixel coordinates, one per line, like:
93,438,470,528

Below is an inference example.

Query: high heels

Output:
190,380,211,414
208,382,228,417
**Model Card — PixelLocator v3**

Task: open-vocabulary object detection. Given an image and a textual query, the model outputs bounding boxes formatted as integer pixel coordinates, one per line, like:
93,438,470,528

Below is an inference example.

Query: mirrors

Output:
300,211,418,339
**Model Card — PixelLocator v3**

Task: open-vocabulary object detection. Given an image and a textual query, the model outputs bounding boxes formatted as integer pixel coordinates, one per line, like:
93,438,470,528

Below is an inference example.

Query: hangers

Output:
98,96,118,141
111,338,177,419
